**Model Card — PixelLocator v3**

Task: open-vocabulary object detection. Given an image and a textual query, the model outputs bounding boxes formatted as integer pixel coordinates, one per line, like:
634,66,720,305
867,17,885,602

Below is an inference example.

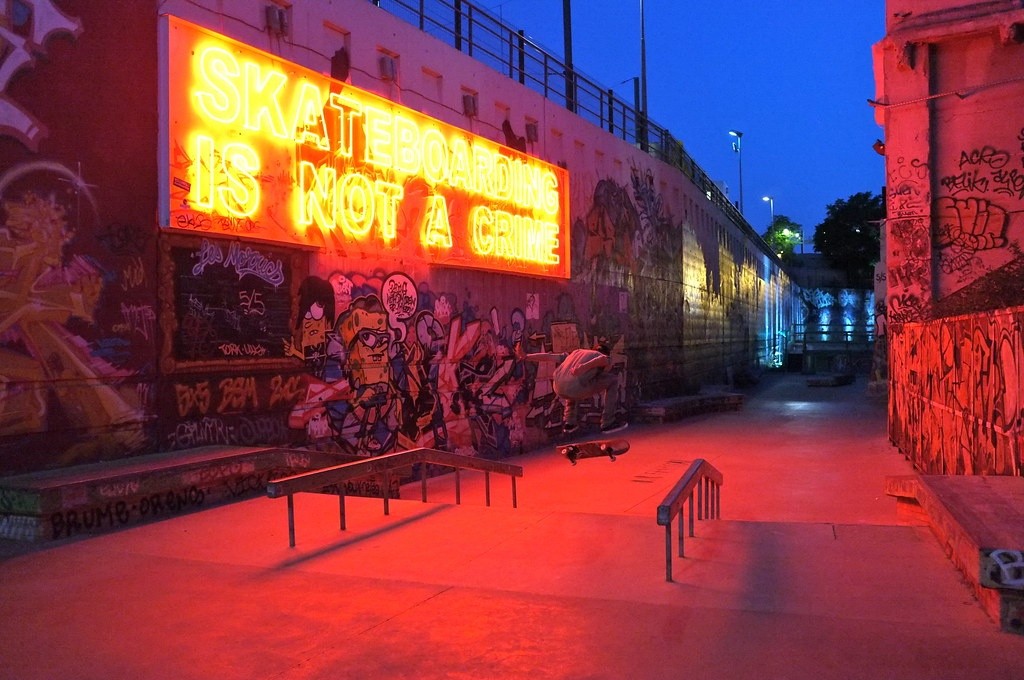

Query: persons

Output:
515,342,628,435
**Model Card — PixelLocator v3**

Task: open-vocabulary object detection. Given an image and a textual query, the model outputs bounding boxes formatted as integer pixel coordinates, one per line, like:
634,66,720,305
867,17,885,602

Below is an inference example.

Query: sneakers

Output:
563,423,580,433
602,419,629,434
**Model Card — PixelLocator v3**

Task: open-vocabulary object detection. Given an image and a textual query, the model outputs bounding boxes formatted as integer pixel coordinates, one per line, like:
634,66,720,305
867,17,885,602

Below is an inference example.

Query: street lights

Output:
762,195,775,251
729,129,743,215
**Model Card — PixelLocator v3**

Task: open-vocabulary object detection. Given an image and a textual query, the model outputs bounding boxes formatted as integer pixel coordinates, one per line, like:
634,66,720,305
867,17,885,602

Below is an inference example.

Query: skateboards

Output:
556,439,633,466
